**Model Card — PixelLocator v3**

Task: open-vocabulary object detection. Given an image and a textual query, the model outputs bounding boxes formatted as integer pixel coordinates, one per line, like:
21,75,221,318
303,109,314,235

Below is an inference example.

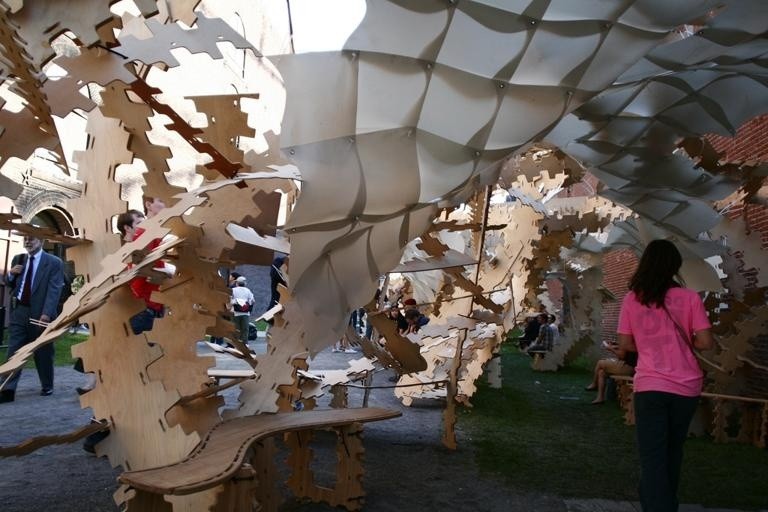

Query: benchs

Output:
117,403,406,496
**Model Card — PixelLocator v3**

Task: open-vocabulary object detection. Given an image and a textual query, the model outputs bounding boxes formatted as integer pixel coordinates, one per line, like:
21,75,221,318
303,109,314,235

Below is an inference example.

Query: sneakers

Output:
584,382,604,405
0,389,16,404
41,387,54,395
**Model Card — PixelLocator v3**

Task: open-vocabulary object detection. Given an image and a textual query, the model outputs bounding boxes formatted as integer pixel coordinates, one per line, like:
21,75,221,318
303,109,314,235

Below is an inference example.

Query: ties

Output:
20,254,35,307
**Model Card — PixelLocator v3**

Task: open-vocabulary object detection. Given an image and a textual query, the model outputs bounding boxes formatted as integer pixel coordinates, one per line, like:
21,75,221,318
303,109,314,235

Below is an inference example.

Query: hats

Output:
404,298,416,305
233,277,246,283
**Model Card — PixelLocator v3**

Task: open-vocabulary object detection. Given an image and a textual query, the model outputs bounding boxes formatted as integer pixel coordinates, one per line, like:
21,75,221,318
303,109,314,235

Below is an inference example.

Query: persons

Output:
84,191,173,455
0,225,63,404
265,258,288,341
585,333,636,407
619,239,714,512
206,272,255,350
390,299,429,337
515,305,558,356
330,279,389,354
